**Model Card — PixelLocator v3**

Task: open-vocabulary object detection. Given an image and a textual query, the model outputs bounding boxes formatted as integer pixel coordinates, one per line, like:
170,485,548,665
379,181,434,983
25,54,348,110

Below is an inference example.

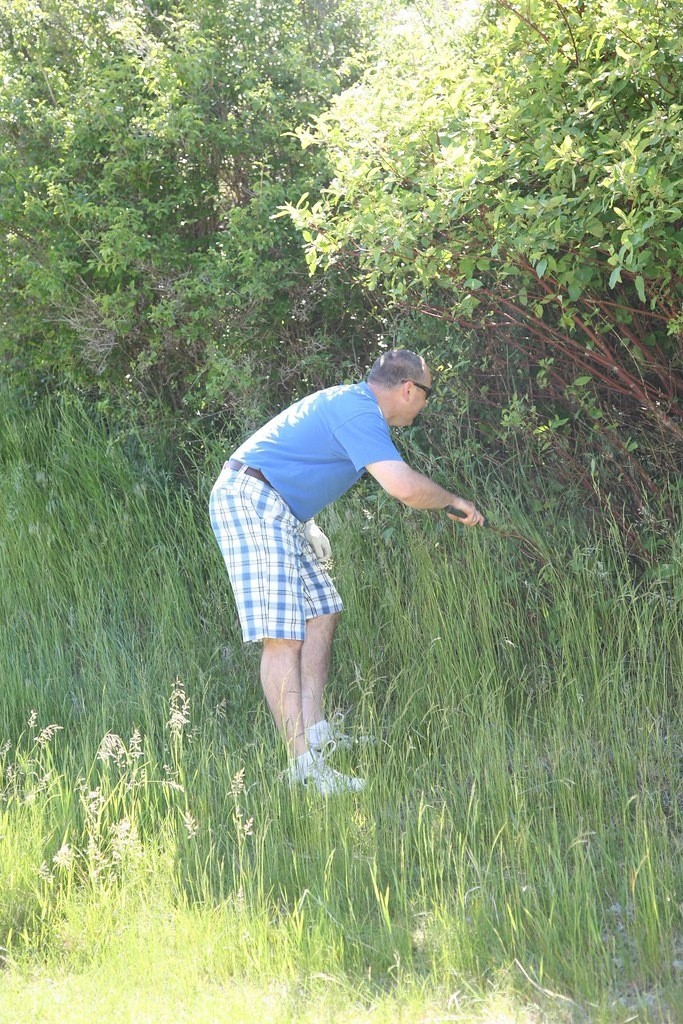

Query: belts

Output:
225,458,269,485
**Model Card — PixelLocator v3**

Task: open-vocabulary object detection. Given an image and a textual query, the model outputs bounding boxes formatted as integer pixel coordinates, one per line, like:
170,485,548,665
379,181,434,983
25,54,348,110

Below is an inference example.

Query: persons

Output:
208,349,487,792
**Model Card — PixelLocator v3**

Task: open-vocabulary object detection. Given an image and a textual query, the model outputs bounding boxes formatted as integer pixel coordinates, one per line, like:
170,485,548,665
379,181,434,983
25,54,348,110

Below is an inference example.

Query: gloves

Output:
305,518,331,562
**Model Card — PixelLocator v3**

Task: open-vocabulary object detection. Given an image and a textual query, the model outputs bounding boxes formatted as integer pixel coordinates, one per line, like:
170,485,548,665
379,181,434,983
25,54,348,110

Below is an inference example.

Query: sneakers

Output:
305,711,375,751
286,740,368,796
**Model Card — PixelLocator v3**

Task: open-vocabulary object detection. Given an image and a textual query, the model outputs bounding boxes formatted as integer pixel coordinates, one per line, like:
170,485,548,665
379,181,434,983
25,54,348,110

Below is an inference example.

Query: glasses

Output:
402,379,434,399
